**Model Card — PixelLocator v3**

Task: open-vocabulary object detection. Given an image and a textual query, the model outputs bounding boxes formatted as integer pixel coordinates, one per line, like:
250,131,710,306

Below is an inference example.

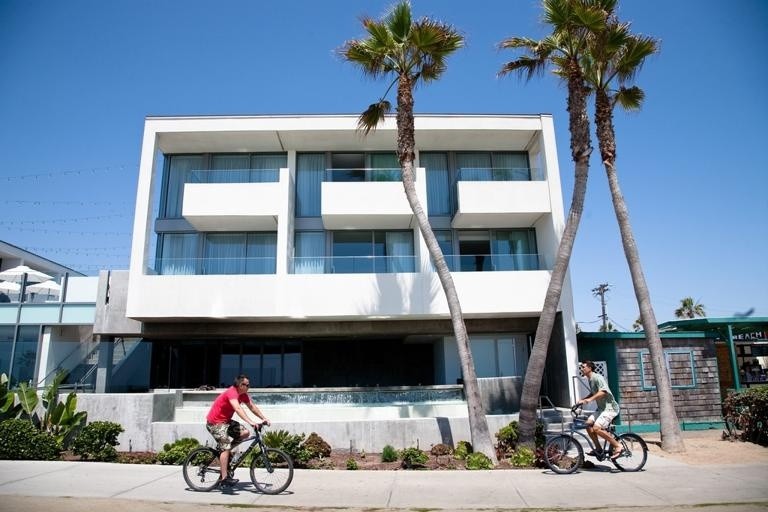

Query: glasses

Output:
242,382,250,386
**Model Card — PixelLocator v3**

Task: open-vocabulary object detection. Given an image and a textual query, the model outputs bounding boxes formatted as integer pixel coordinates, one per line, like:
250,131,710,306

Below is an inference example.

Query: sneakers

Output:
219,477,239,490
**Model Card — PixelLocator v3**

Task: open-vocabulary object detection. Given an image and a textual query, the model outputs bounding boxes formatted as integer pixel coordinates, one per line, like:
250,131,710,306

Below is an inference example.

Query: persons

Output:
575,359,626,461
206,374,271,495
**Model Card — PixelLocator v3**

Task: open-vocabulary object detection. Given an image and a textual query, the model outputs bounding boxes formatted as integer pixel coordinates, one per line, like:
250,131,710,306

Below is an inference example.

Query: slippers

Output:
610,448,626,460
586,448,603,456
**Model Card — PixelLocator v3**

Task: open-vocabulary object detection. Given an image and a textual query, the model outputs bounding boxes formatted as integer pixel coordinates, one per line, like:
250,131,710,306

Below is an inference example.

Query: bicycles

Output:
177,416,295,496
542,403,650,476
